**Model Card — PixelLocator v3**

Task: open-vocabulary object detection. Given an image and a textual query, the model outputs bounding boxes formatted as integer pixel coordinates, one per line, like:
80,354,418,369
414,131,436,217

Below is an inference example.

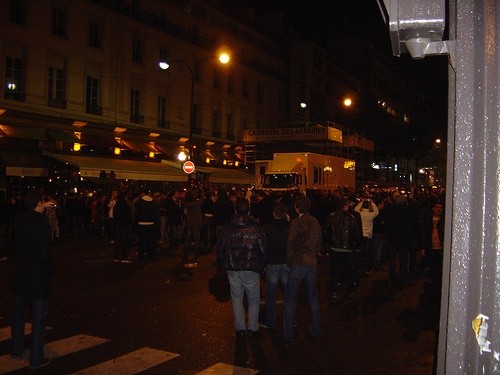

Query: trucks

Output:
257,152,355,193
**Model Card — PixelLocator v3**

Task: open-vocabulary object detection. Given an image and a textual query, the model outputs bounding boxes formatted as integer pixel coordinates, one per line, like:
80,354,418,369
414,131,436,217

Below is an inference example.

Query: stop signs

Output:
183,161,195,174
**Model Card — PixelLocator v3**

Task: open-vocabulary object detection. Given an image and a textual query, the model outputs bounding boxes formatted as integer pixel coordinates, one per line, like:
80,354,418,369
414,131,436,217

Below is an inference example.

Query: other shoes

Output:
10,355,50,368
304,331,319,341
234,329,260,338
259,320,274,329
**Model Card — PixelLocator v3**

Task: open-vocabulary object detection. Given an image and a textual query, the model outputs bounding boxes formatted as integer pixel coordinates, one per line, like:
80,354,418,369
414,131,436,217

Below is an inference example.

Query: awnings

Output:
0,151,256,184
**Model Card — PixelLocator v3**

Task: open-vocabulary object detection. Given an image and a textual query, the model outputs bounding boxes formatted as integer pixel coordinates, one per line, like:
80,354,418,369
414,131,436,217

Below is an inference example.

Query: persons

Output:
258,203,298,329
6,190,55,369
282,196,321,341
0,182,446,306
216,199,268,339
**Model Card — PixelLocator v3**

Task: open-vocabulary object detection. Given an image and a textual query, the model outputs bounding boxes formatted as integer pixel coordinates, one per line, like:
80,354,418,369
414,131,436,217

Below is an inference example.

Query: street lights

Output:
300,96,353,154
159,51,232,186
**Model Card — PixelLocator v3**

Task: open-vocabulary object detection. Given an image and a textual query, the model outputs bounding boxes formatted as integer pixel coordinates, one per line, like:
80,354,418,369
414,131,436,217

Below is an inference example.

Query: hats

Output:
234,200,250,217
